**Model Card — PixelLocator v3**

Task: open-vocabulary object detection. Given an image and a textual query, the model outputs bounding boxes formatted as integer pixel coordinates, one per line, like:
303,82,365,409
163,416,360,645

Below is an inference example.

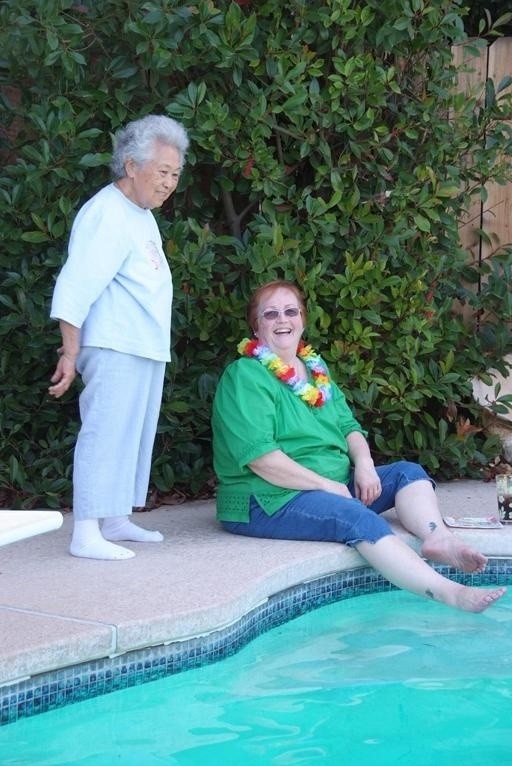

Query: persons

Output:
210,280,508,615
48,115,190,560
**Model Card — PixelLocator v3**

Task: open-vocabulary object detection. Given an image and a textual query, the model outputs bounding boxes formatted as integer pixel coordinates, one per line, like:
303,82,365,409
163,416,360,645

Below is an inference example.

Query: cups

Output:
496,473,512,525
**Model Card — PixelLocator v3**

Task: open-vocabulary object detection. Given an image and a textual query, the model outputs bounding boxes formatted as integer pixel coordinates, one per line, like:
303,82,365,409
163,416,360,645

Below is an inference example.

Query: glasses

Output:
255,307,302,320
